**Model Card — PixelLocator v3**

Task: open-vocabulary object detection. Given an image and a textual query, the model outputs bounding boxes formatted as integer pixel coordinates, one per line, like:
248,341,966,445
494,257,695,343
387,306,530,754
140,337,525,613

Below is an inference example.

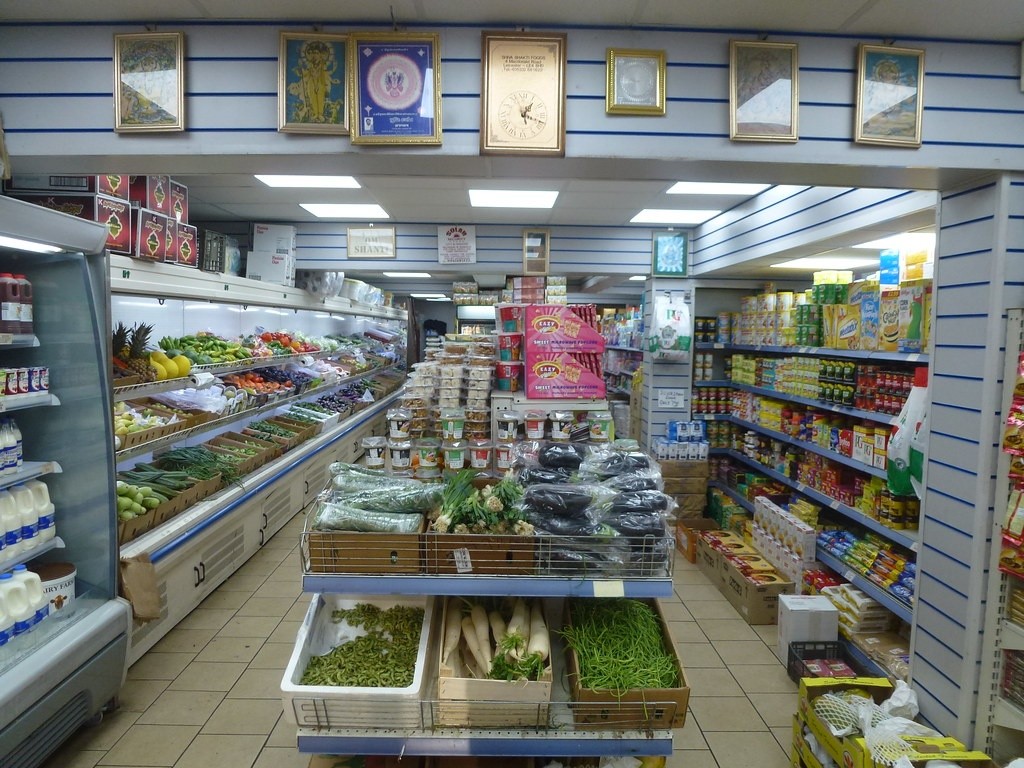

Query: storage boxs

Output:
562,597,690,728
657,459,849,626
199,229,226,273
339,279,382,305
3,171,200,267
440,596,554,726
281,590,440,728
310,479,536,574
823,279,934,354
246,223,298,287
778,594,839,668
791,677,998,768
630,391,643,446
113,353,405,544
787,641,870,686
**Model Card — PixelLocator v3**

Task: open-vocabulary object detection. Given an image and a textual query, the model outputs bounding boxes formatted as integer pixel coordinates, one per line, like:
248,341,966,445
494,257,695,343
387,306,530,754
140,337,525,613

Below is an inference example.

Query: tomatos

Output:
225,372,292,393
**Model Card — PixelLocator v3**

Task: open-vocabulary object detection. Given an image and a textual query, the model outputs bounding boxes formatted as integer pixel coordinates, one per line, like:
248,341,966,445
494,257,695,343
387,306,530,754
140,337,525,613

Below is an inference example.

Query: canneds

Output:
880,488,920,530
0,367,49,396
712,281,851,347
816,356,915,416
691,351,737,412
743,430,758,458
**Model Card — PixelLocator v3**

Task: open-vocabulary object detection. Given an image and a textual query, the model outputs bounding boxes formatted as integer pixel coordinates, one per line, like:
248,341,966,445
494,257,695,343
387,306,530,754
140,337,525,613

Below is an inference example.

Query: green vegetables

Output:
218,401,335,465
154,445,246,491
548,597,680,721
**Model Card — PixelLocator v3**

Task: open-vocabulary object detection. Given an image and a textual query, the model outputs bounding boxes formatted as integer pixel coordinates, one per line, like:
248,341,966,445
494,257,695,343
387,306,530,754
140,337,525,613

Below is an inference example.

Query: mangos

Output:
113,401,155,435
159,334,251,363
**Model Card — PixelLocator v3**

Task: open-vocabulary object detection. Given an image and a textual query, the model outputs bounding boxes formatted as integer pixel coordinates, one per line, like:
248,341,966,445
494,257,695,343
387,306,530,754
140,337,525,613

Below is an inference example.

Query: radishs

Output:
443,595,550,679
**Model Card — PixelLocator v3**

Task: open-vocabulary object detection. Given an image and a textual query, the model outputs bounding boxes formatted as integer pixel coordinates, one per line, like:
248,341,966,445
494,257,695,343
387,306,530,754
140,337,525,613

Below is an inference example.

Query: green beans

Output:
297,602,424,687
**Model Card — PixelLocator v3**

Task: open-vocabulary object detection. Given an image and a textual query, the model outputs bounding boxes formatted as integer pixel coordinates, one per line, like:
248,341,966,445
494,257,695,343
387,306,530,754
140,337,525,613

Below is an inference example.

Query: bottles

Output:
12,565,49,625
0,418,23,478
0,573,37,636
0,591,16,647
0,479,56,564
0,272,34,336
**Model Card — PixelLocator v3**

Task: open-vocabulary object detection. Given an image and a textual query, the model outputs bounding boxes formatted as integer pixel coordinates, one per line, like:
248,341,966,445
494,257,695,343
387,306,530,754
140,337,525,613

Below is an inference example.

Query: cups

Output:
416,437,440,466
499,306,522,331
387,438,412,470
469,439,494,473
496,361,523,392
385,408,413,438
361,436,387,470
498,332,523,360
439,406,465,439
413,466,444,484
523,410,547,441
496,440,514,473
441,439,469,472
495,410,521,443
586,411,613,442
548,410,575,441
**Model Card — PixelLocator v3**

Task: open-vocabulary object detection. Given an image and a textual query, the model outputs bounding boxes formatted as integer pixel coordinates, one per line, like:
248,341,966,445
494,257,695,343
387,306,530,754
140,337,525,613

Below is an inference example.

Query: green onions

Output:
428,466,535,536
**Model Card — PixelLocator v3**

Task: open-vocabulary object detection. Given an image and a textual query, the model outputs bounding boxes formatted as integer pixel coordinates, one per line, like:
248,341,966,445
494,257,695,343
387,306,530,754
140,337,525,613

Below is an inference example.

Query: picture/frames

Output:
276,28,352,136
651,230,689,279
853,43,927,151
113,30,186,133
347,226,396,259
727,37,802,145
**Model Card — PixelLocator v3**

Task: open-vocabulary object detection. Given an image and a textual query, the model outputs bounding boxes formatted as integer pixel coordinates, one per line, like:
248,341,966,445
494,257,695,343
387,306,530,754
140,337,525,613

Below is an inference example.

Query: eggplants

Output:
253,366,311,385
315,389,361,413
511,441,673,575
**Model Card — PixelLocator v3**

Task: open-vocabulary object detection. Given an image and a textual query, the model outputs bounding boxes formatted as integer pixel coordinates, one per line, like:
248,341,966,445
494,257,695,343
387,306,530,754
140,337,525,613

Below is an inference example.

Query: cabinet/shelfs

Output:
604,345,643,440
297,573,675,757
693,343,929,688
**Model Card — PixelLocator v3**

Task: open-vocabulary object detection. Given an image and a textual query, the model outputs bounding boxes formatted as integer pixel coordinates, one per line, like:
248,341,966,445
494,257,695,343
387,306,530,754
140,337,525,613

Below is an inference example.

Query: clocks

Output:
606,47,666,117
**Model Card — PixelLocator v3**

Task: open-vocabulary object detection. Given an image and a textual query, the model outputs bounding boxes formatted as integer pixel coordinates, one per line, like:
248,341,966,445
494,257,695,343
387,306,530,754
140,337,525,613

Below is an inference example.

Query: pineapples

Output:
109,320,157,383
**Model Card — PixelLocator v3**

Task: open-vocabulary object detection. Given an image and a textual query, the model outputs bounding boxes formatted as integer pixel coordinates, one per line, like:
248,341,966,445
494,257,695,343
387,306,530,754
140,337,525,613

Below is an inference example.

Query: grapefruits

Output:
147,349,190,380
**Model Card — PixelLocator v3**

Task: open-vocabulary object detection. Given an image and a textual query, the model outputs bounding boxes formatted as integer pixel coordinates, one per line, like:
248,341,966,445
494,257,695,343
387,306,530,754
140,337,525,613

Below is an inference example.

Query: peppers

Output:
260,331,319,359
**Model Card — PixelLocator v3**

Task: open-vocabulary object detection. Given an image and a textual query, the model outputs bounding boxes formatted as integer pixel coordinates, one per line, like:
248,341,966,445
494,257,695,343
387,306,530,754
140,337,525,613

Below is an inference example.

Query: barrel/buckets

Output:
42,564,76,619
